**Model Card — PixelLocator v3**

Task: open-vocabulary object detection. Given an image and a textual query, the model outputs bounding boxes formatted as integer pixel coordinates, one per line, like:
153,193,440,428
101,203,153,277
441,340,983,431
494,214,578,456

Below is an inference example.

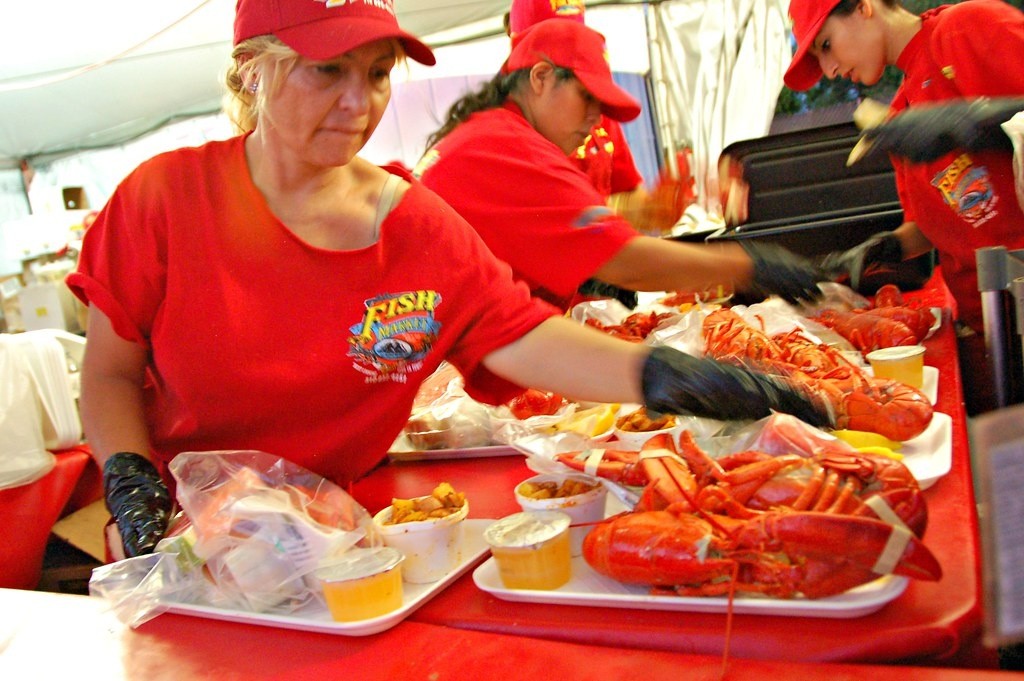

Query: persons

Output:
403,0,827,314
64,0,831,560
784,0,1023,410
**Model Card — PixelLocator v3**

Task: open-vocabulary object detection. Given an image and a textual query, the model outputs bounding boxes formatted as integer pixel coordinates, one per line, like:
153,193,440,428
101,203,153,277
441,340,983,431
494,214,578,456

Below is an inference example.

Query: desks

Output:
341,288,985,664
0,584,1024,680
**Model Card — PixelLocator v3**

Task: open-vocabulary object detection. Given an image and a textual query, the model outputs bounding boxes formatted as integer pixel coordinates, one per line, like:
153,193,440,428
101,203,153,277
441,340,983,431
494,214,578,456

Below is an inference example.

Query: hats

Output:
783,0,840,92
502,18,641,124
507,0,583,39
233,0,436,66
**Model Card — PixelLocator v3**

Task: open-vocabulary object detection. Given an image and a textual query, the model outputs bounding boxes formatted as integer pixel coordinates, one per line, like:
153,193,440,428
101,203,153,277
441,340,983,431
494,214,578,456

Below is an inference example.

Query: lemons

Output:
826,429,907,462
540,401,620,437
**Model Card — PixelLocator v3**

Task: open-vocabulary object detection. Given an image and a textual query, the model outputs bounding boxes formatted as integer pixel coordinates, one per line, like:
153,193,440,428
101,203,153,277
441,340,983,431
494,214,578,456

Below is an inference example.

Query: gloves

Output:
820,231,903,291
102,451,171,558
730,237,824,307
577,277,637,309
864,95,1023,163
642,345,835,432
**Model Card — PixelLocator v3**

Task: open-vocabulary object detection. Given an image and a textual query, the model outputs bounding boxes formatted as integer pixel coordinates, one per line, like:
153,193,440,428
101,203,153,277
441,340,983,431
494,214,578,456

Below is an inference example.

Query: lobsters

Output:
504,283,943,681
